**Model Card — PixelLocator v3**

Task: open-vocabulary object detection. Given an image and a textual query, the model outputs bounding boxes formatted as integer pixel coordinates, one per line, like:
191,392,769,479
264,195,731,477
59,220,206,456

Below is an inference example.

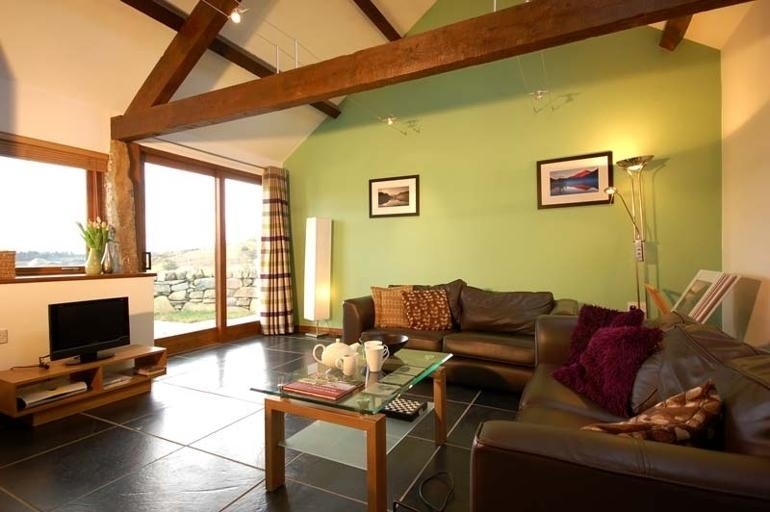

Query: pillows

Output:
458,287,553,336
552,326,660,415
575,379,725,451
370,287,452,330
562,304,645,362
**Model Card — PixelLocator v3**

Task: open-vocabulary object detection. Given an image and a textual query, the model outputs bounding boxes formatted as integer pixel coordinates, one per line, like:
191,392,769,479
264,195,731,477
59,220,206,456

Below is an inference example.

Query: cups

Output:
364,340,390,373
335,352,360,376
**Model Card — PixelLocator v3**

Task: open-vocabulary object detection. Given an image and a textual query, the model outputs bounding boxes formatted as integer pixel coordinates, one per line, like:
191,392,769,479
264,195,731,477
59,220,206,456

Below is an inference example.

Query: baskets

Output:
0,252,17,280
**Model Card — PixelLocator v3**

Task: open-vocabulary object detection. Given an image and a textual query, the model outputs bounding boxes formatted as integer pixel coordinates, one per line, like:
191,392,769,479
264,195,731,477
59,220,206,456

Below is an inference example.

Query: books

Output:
103,373,131,390
133,364,165,377
283,378,364,405
670,268,739,323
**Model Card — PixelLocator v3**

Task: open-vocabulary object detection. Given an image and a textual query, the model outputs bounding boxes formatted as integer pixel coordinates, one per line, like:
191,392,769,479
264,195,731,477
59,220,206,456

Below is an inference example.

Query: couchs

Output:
468,314,769,512
343,286,579,397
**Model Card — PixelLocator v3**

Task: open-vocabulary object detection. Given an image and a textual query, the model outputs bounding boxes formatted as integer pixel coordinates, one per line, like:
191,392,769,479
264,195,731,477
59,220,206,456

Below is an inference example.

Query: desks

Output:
0,345,167,426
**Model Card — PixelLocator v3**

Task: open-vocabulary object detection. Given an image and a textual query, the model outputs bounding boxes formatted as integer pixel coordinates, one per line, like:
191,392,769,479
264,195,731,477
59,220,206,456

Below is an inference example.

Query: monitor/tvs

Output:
47,296,131,366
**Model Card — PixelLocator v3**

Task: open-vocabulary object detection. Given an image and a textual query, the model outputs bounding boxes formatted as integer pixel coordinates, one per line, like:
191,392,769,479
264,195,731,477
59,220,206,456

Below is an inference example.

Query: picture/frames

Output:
536,151,613,209
668,268,740,325
369,176,419,218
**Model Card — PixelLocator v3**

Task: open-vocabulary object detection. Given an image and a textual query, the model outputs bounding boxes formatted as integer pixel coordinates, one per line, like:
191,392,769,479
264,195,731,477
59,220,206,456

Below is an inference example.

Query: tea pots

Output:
313,338,362,369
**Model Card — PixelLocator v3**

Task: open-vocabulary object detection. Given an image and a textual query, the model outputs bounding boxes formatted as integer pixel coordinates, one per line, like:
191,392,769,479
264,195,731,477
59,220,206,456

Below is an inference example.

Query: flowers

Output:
80,220,107,248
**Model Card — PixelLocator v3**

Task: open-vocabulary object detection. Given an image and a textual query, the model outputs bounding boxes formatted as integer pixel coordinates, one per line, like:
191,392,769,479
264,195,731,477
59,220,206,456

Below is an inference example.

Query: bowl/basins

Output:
359,333,410,358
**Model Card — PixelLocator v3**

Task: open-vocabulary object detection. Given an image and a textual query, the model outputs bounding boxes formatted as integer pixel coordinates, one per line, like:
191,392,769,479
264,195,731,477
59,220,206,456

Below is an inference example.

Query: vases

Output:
102,242,121,274
104,224,115,244
85,250,101,276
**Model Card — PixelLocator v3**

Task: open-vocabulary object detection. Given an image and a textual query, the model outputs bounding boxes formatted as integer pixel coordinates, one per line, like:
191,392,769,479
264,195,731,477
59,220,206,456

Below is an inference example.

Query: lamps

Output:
606,154,654,310
304,217,332,338
